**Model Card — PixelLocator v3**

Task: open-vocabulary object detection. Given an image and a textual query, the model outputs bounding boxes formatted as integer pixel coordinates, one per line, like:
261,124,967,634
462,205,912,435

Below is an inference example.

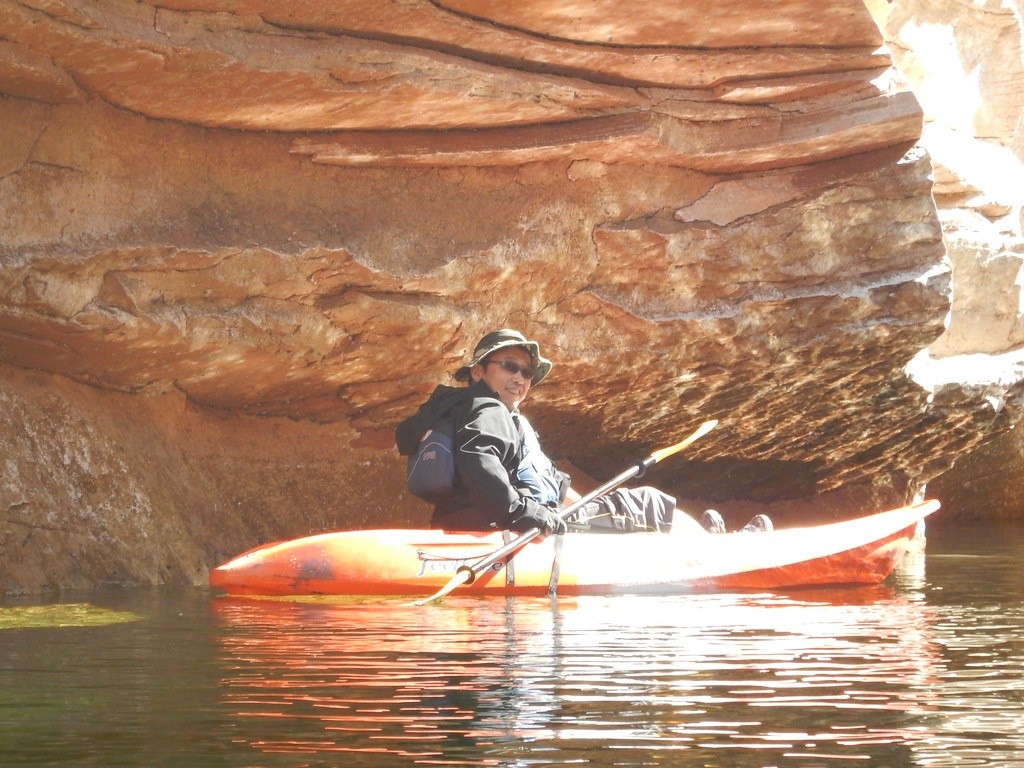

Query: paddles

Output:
415,419,721,606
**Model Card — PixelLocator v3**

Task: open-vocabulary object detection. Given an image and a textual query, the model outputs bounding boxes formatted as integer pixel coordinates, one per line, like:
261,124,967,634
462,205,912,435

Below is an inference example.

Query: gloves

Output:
532,506,567,536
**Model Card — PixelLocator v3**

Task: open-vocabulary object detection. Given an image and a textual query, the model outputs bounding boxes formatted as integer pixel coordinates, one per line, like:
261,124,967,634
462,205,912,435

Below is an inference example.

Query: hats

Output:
455,329,552,389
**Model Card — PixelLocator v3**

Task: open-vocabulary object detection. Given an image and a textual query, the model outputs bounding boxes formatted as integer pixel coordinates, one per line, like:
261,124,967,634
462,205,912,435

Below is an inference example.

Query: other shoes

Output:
738,514,773,533
703,509,726,534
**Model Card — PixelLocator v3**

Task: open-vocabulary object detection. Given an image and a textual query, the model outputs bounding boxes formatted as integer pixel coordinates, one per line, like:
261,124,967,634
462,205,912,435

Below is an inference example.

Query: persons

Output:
395,329,774,534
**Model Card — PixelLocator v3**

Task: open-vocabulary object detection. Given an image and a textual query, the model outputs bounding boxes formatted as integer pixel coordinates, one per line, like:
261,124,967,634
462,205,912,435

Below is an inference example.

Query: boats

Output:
210,499,948,597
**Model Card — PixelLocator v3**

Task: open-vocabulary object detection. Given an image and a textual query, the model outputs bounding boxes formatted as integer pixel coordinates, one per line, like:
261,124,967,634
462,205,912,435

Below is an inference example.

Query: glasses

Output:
484,360,535,380
